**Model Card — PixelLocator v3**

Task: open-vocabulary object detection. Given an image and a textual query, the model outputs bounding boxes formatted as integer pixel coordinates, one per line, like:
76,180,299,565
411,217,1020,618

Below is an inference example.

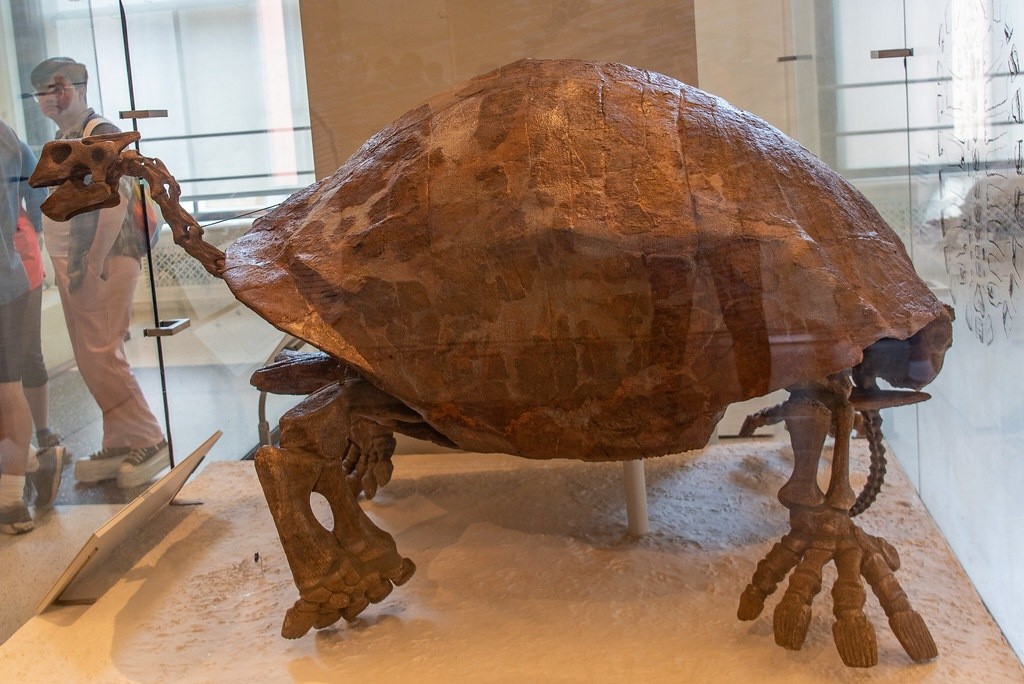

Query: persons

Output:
32,57,173,490
0,119,48,539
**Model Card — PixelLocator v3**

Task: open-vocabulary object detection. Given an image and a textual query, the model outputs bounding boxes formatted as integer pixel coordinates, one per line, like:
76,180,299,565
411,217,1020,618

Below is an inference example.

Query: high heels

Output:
75,446,127,482
117,439,171,489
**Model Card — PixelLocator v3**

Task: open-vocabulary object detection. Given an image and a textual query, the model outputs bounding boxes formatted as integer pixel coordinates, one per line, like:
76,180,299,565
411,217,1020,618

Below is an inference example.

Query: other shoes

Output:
27,445,66,510
0,506,34,534
37,427,67,457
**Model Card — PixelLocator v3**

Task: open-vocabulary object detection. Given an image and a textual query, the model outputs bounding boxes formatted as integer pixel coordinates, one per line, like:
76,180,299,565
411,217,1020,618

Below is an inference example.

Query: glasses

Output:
31,81,86,102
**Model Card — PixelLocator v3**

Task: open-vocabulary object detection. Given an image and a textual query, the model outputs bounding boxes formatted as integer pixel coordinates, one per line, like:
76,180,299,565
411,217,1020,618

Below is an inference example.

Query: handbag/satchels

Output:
82,116,165,250
